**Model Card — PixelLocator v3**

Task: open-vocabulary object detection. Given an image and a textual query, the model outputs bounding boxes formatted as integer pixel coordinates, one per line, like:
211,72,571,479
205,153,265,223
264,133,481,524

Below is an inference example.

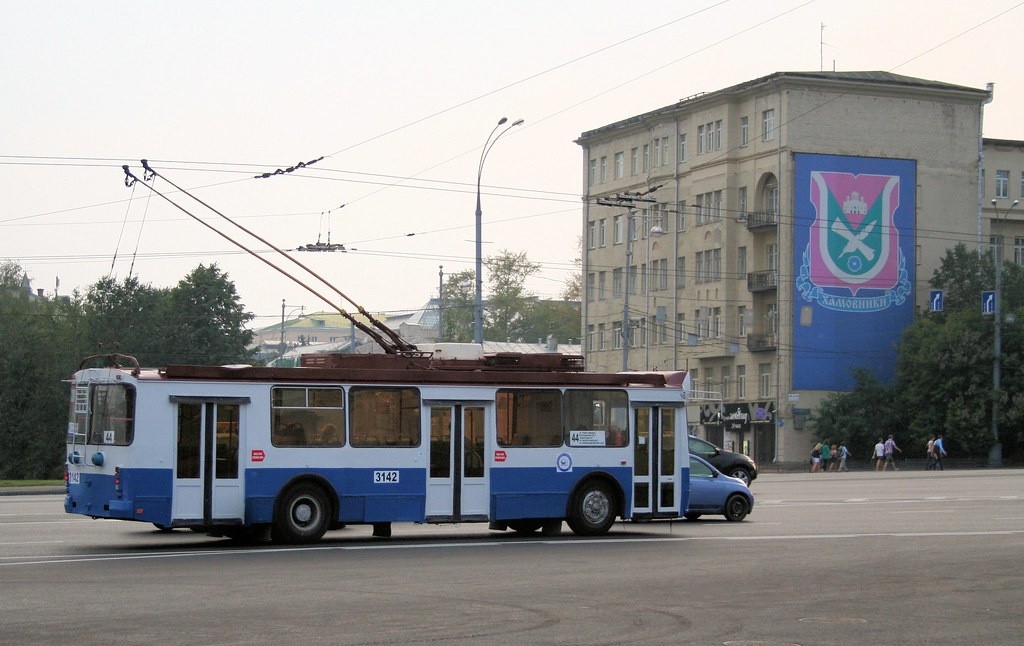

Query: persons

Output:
925,435,947,471
872,435,901,472
810,438,852,473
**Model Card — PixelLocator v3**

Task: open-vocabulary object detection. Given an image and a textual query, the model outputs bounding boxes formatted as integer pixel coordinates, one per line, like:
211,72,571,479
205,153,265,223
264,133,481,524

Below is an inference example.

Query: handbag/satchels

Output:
934,445,939,454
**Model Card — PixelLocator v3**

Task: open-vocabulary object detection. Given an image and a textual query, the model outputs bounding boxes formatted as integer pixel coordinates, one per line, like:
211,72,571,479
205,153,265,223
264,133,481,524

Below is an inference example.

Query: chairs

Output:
94,417,559,466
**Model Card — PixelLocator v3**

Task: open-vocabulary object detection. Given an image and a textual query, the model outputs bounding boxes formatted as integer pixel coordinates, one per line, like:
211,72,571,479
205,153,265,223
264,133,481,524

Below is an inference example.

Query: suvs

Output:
637,430,759,488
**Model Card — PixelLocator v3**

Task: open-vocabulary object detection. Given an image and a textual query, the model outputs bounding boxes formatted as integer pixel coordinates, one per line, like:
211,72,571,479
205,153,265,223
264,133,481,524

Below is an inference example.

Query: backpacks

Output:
837,446,844,457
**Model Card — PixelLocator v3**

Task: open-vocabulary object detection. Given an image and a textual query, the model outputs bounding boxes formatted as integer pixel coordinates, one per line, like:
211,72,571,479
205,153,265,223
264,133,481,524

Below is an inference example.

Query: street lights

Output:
474,116,524,345
622,208,665,372
280,298,305,356
990,199,1021,464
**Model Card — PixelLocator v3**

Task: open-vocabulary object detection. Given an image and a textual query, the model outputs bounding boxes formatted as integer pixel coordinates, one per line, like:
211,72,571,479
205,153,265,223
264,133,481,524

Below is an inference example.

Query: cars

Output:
631,452,754,524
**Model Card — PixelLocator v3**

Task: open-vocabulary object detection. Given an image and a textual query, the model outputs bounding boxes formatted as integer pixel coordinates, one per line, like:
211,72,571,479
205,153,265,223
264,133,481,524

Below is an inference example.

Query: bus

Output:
62,158,729,547
